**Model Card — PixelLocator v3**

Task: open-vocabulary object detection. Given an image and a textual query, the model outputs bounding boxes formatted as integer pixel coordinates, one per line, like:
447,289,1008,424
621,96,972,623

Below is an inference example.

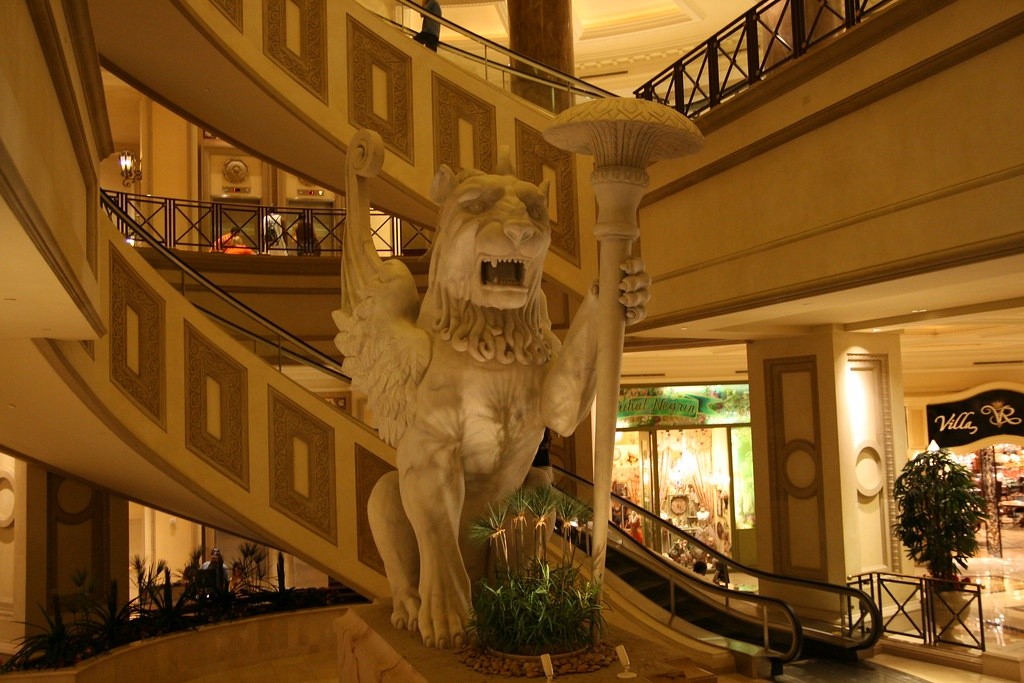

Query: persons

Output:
292,212,321,256
261,204,287,257
413,0,442,52
209,227,255,255
199,547,229,590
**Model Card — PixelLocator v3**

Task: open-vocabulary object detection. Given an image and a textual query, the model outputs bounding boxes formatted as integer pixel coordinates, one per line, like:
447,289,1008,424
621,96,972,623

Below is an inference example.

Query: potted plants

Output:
893,448,992,647
462,483,613,661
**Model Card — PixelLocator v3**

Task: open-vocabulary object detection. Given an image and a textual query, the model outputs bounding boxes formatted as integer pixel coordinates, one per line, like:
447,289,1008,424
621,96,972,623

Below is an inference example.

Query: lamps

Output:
118,148,143,188
